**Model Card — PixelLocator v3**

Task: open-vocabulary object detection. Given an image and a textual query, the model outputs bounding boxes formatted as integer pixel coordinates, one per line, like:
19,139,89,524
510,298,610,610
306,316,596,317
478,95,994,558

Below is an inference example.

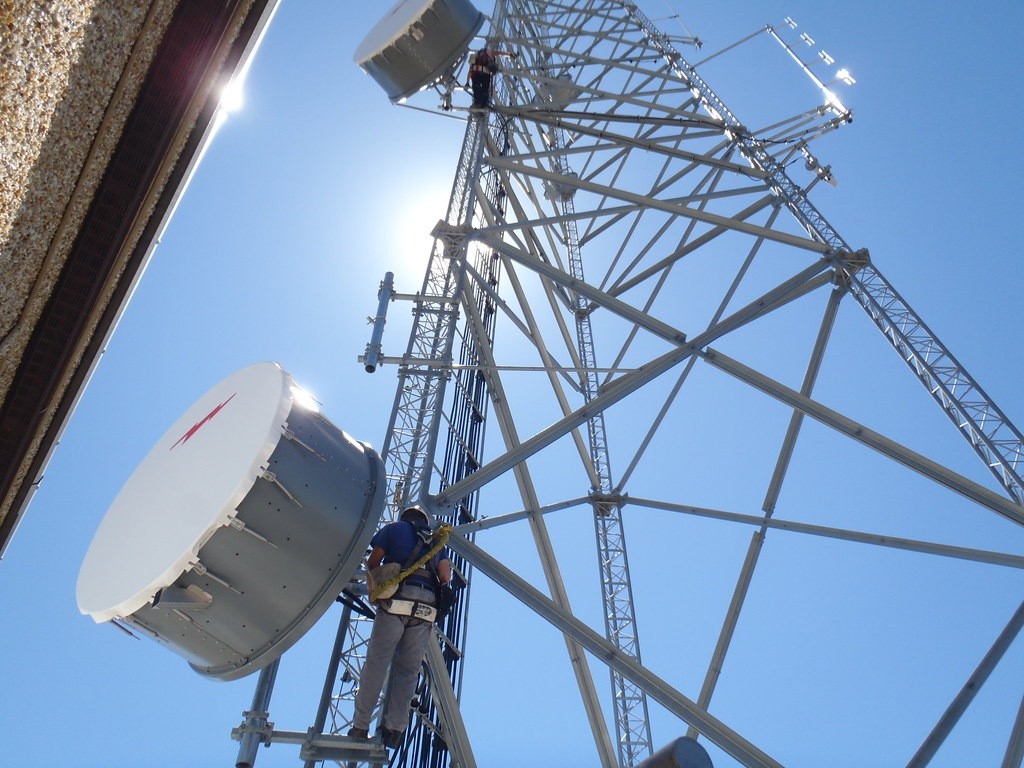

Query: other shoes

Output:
376,726,401,749
348,727,368,739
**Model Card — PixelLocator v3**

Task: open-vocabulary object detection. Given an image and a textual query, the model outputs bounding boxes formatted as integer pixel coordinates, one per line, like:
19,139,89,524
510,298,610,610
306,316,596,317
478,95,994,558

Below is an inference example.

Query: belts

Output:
406,581,434,593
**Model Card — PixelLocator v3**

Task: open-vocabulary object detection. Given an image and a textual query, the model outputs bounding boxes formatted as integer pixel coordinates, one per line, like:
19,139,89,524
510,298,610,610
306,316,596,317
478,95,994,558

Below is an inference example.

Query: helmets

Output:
400,505,430,526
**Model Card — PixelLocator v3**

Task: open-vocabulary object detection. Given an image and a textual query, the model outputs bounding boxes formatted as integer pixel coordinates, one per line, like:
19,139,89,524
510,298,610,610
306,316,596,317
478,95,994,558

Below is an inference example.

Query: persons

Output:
465,49,514,108
347,504,451,750
